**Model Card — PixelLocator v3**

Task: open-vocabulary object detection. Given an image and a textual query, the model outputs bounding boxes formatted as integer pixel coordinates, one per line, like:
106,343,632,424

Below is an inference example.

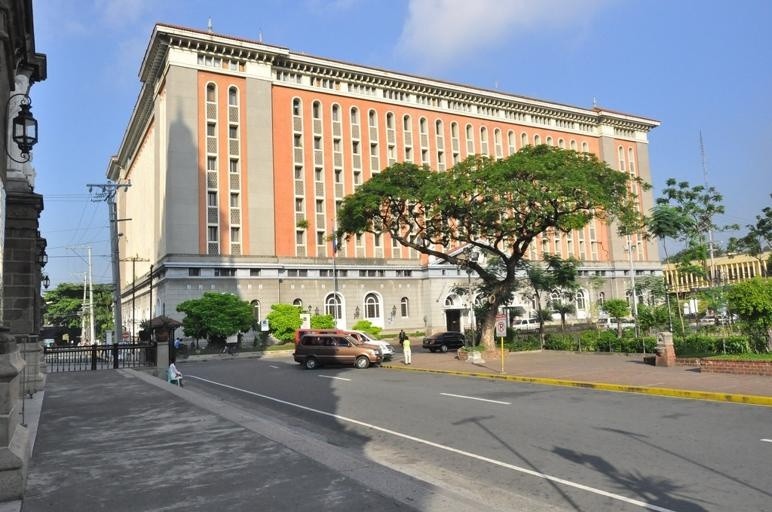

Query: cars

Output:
686,311,740,327
512,317,540,334
596,316,638,332
422,331,465,353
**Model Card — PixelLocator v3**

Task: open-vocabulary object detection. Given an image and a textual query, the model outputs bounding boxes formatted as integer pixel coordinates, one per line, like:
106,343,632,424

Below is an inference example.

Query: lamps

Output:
37,245,49,268
40,273,51,290
0,92,39,166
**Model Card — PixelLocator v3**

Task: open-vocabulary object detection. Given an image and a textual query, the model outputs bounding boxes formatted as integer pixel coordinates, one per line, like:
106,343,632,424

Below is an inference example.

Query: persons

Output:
179,338,187,345
110,330,116,356
174,336,181,349
399,329,405,348
122,326,128,340
168,358,184,387
401,336,412,365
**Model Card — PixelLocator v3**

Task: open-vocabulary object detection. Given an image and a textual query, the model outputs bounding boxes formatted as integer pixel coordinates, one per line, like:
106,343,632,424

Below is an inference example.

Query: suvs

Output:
292,333,384,370
295,328,396,362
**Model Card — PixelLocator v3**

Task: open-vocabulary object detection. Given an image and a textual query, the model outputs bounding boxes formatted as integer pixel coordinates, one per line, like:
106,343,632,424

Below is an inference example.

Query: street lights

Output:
624,233,641,338
